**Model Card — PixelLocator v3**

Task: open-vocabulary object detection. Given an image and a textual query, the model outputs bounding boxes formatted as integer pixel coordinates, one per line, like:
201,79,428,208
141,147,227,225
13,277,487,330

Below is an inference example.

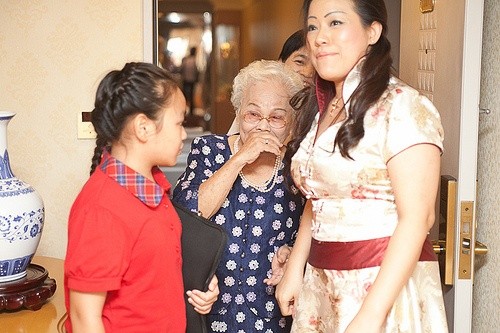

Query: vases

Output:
0,111,46,283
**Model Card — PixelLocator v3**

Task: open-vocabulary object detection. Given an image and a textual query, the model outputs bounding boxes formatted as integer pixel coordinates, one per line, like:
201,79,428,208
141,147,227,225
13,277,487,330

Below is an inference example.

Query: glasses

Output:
239,112,291,128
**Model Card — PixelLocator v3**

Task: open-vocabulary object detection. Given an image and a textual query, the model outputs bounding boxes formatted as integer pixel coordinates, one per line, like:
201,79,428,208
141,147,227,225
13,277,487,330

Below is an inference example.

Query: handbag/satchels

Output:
171,201,225,333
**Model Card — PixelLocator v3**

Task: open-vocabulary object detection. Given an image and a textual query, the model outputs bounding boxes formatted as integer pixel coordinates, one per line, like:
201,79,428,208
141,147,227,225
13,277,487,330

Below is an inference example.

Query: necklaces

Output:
330,96,339,114
233,136,281,193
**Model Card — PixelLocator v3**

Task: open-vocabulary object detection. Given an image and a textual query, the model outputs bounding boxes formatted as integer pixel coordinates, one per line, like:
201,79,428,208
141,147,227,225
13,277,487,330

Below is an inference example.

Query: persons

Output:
275,0,449,333
180,47,199,114
64,62,220,333
227,28,317,136
172,59,307,333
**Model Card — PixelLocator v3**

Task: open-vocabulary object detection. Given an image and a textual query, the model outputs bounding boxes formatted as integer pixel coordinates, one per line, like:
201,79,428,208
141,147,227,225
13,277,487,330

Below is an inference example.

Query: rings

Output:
266,139,268,144
